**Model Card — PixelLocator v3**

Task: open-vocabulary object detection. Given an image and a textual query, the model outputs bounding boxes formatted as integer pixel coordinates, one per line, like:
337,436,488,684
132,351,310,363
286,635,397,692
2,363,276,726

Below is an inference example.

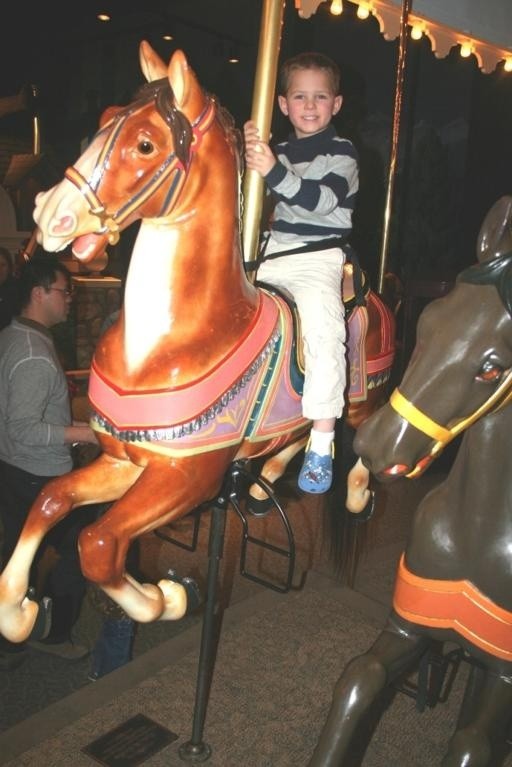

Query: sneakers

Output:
25,630,90,661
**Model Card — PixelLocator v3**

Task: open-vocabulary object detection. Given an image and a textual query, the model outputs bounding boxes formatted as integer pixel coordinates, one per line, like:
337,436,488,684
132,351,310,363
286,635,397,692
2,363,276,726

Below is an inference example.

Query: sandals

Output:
296,430,342,496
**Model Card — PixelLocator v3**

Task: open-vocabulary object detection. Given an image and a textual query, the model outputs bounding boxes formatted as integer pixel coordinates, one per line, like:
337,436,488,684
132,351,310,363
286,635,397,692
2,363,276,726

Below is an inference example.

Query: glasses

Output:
44,284,77,299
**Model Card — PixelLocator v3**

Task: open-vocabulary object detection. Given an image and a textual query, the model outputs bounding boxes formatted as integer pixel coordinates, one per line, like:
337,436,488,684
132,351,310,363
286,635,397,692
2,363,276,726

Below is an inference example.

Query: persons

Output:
0,255,102,664
239,45,365,498
